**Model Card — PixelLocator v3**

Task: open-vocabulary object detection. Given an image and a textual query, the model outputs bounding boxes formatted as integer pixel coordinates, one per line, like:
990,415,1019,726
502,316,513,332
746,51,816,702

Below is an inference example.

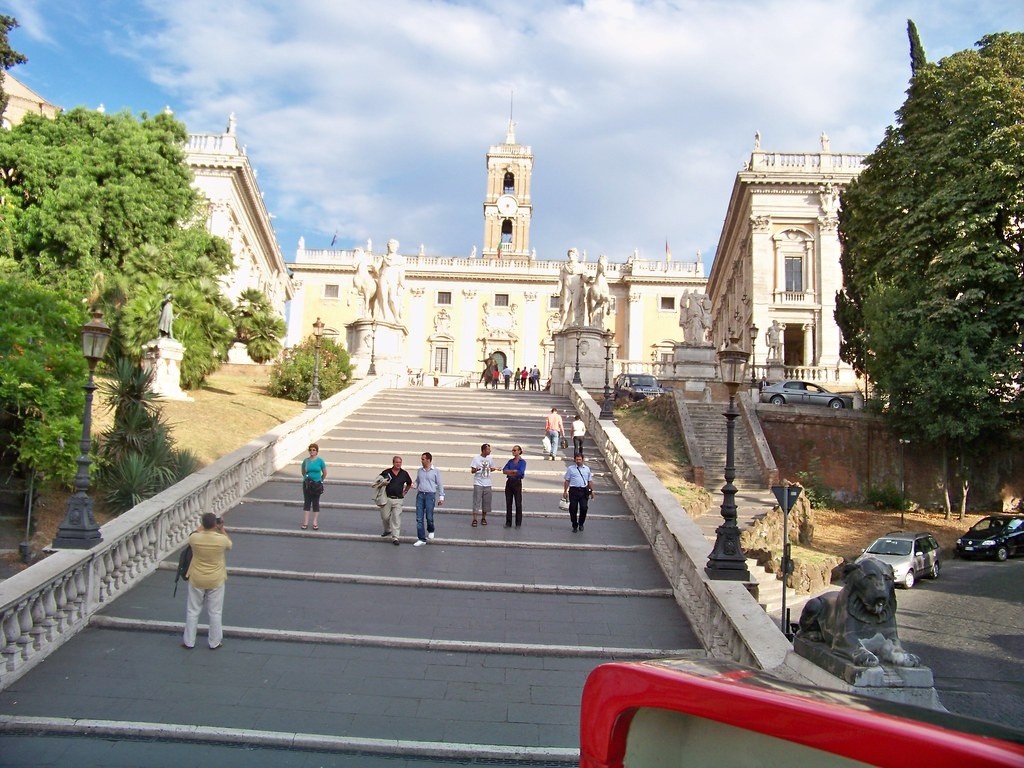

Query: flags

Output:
665,240,671,268
331,234,336,246
497,242,502,258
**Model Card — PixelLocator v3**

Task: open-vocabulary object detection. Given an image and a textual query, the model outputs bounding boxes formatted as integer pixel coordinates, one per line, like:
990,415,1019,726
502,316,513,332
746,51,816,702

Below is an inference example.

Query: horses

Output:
586,255,617,326
347,247,377,319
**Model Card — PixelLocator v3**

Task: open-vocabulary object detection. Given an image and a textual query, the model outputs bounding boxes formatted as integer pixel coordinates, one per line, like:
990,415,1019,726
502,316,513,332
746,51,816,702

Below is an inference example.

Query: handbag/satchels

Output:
560,436,568,449
305,477,324,496
559,496,570,511
586,485,593,493
175,545,193,582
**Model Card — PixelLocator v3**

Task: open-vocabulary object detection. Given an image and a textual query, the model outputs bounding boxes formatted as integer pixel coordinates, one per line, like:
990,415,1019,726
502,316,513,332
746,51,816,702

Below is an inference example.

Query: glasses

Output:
511,449,517,452
485,444,490,447
309,449,316,452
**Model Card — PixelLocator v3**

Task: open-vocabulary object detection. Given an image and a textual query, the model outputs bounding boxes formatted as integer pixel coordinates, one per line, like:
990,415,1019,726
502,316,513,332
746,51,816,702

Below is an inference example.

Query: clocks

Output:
496,194,520,216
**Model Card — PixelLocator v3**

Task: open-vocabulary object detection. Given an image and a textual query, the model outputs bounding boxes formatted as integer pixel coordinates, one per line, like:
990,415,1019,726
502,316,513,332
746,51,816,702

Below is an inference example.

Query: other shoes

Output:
218,642,223,648
579,524,584,531
381,531,391,537
472,519,477,526
481,519,487,525
515,525,521,529
573,527,577,533
503,523,509,528
393,540,400,545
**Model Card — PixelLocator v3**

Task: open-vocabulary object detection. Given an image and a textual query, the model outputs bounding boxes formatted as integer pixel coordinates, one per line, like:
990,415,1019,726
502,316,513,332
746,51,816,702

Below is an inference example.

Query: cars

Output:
760,380,853,409
955,516,1024,562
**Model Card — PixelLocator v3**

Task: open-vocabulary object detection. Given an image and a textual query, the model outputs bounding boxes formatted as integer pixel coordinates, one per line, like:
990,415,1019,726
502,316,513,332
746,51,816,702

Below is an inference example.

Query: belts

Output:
387,495,402,499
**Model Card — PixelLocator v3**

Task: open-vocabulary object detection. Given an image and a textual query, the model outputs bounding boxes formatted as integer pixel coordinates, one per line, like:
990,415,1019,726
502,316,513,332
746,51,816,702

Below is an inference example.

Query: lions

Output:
799,558,921,669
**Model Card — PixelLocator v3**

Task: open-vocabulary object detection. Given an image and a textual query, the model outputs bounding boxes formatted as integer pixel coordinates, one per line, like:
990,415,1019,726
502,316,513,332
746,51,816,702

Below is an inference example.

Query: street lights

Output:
704,338,751,580
599,329,616,420
749,324,759,389
305,317,325,409
366,319,379,375
51,308,113,550
572,328,582,384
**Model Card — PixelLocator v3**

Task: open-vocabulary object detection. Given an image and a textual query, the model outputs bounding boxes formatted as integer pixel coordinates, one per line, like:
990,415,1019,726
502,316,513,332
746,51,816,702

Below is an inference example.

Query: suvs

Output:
613,373,665,403
854,530,944,588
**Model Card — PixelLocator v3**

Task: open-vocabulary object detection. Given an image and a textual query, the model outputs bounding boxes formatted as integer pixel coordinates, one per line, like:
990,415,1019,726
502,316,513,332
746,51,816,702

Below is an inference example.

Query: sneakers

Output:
413,539,427,546
427,532,435,539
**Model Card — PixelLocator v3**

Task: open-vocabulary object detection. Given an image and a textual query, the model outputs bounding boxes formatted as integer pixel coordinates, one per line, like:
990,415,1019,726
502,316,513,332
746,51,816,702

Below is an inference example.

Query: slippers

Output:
301,524,309,529
313,523,319,530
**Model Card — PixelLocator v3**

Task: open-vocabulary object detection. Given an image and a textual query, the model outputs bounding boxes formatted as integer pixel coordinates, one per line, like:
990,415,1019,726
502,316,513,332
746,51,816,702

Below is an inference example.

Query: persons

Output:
570,415,586,462
180,513,232,649
753,129,759,152
563,454,594,533
405,367,439,386
470,444,526,529
743,161,747,171
545,408,564,461
381,452,445,547
159,292,173,338
296,236,639,264
477,352,498,383
492,365,541,391
93,103,237,134
301,444,327,530
376,238,404,324
557,248,590,330
820,131,830,153
765,319,787,360
758,376,770,393
697,249,703,262
680,288,712,342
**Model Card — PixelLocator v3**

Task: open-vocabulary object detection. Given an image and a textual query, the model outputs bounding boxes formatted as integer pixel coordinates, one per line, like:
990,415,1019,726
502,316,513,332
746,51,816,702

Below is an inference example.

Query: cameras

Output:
217,517,220,524
